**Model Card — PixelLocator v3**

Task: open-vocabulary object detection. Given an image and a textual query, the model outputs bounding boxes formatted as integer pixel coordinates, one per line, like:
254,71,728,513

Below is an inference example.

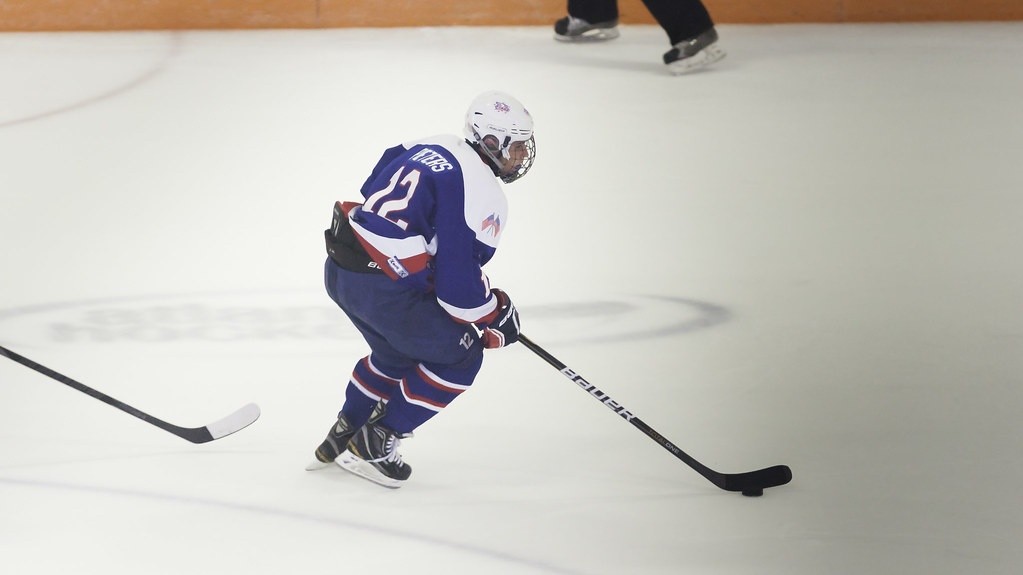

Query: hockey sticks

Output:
0,345,262,444
515,333,792,492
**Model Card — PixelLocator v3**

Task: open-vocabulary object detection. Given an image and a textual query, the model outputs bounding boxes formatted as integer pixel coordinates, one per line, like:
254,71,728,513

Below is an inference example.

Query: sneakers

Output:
305,411,357,471
334,397,412,488
663,26,727,73
554,15,618,40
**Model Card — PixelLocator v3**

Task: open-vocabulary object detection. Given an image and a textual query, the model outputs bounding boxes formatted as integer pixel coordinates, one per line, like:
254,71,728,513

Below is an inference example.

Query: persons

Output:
553,0,728,75
307,91,537,489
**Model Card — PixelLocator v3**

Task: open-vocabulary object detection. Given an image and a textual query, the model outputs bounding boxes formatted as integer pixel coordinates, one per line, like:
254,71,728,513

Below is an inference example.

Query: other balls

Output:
742,490,763,496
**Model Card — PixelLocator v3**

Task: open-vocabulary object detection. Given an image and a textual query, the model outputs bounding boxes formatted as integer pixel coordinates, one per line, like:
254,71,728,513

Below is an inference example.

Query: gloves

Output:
469,288,520,349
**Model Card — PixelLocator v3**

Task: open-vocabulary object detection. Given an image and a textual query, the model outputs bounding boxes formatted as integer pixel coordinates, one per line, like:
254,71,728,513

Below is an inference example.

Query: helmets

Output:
464,90,537,184
